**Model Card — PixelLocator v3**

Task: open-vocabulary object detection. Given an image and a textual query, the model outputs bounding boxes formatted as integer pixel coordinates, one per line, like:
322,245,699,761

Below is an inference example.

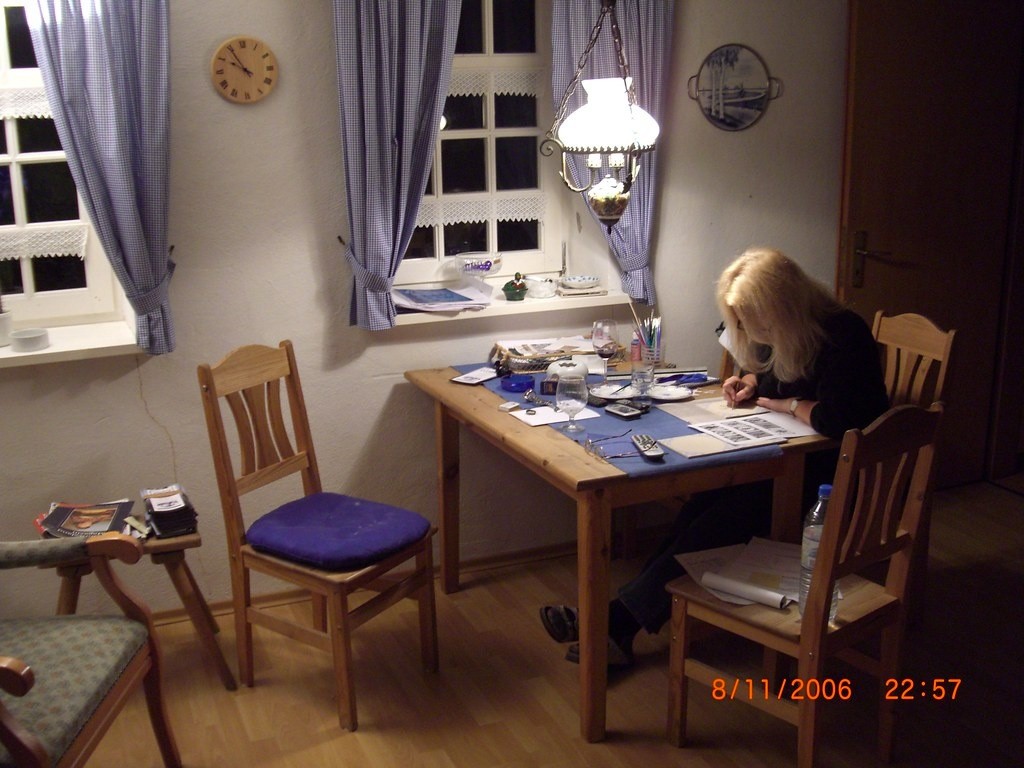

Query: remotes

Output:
630,433,665,461
615,399,651,413
605,405,642,420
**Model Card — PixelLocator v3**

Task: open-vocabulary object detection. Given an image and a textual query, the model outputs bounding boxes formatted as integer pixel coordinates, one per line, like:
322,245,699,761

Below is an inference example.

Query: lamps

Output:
540,0,659,235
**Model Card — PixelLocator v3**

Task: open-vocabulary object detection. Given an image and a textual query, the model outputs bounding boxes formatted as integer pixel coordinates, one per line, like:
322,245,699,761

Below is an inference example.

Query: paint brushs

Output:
625,293,661,346
610,383,631,395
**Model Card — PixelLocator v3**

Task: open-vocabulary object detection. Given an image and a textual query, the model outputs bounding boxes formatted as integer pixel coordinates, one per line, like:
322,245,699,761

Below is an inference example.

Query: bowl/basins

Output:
526,279,557,299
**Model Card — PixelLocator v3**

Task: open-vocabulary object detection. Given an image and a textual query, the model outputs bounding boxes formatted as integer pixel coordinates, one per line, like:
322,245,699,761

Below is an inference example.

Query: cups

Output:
0,310,14,345
502,287,528,300
631,361,654,386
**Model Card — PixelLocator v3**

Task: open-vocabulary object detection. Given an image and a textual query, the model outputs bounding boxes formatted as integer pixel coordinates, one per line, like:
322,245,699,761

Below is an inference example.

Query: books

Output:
39,480,134,547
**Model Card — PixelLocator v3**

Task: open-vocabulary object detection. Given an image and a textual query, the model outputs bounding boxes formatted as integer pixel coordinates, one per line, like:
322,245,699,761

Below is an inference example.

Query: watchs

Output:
790,398,804,417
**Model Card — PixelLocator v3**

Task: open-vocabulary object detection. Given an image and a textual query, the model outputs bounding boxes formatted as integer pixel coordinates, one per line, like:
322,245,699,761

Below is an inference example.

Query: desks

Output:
405,349,832,741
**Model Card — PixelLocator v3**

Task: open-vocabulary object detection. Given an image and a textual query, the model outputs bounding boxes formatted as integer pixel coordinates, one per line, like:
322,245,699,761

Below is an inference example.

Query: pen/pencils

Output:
732,382,740,409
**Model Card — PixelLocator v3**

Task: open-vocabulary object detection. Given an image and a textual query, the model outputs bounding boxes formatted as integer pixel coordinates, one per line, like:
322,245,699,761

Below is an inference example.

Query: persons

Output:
539,248,891,668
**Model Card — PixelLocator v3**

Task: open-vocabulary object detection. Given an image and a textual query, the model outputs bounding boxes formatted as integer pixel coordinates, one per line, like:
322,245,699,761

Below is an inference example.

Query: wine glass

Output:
556,375,589,433
591,318,618,388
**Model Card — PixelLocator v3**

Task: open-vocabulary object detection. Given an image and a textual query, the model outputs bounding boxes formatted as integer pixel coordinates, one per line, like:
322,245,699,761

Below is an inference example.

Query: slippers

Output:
540,603,574,645
564,635,636,664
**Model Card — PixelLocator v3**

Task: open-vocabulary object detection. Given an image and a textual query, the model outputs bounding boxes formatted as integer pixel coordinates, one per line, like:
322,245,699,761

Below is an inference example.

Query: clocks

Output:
210,36,278,104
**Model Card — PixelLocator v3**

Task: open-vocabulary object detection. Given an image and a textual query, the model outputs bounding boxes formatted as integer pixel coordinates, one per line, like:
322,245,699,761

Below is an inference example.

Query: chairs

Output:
762,312,956,628
0,533,182,768
665,400,950,768
198,340,439,733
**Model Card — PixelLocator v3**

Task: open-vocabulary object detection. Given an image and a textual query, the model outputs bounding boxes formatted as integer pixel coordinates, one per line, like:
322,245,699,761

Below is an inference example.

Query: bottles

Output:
630,331,641,361
798,484,838,620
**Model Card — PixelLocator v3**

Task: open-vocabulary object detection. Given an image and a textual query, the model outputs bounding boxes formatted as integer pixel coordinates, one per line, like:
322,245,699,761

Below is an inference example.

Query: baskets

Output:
494,334,626,374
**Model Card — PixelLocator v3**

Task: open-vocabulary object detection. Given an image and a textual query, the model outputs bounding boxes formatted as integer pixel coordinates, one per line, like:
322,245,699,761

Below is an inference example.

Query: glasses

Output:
715,322,726,337
583,429,658,464
737,320,771,339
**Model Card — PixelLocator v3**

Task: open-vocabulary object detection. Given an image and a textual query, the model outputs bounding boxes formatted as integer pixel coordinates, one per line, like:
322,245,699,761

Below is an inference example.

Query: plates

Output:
590,383,642,398
561,275,600,288
645,385,692,400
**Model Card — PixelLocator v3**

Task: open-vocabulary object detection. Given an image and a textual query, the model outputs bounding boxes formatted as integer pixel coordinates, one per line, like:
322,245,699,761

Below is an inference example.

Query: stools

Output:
37,516,237,689
